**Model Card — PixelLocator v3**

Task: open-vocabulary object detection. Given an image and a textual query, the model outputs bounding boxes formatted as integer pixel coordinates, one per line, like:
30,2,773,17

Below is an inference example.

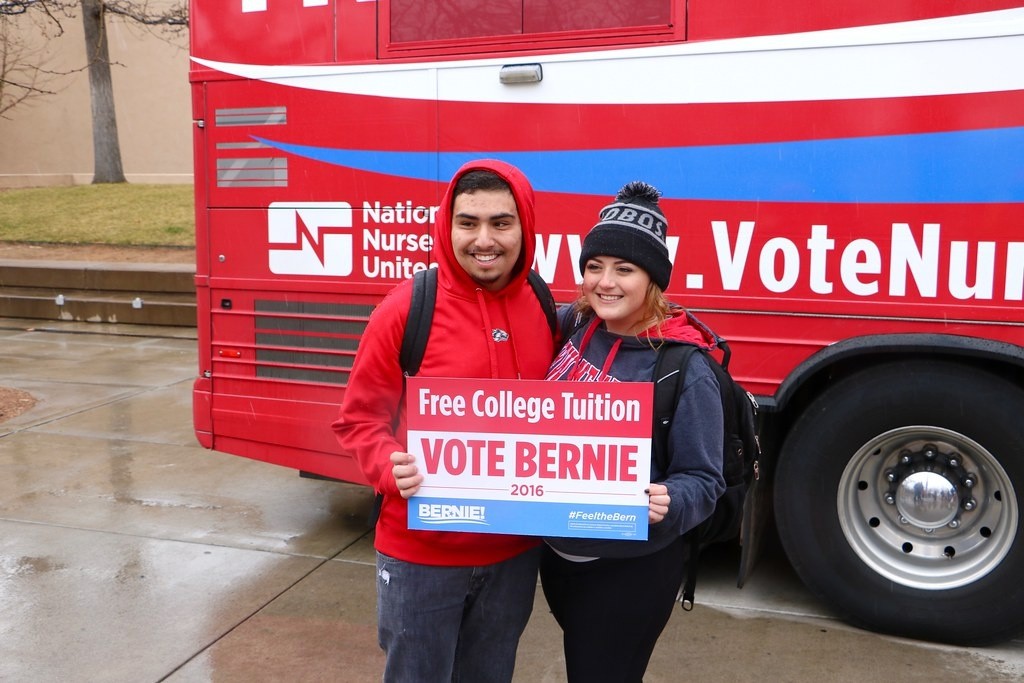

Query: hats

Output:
580,181,672,293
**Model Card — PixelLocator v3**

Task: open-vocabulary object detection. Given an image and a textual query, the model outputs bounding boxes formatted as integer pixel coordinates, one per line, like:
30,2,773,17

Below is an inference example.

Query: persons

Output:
540,180,727,683
330,158,562,682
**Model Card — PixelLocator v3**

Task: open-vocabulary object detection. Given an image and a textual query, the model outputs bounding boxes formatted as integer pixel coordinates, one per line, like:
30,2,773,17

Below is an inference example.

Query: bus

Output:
191,0,1024,647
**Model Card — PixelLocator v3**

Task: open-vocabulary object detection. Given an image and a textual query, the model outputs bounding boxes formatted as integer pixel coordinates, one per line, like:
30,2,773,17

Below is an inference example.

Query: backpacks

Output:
652,340,762,545
